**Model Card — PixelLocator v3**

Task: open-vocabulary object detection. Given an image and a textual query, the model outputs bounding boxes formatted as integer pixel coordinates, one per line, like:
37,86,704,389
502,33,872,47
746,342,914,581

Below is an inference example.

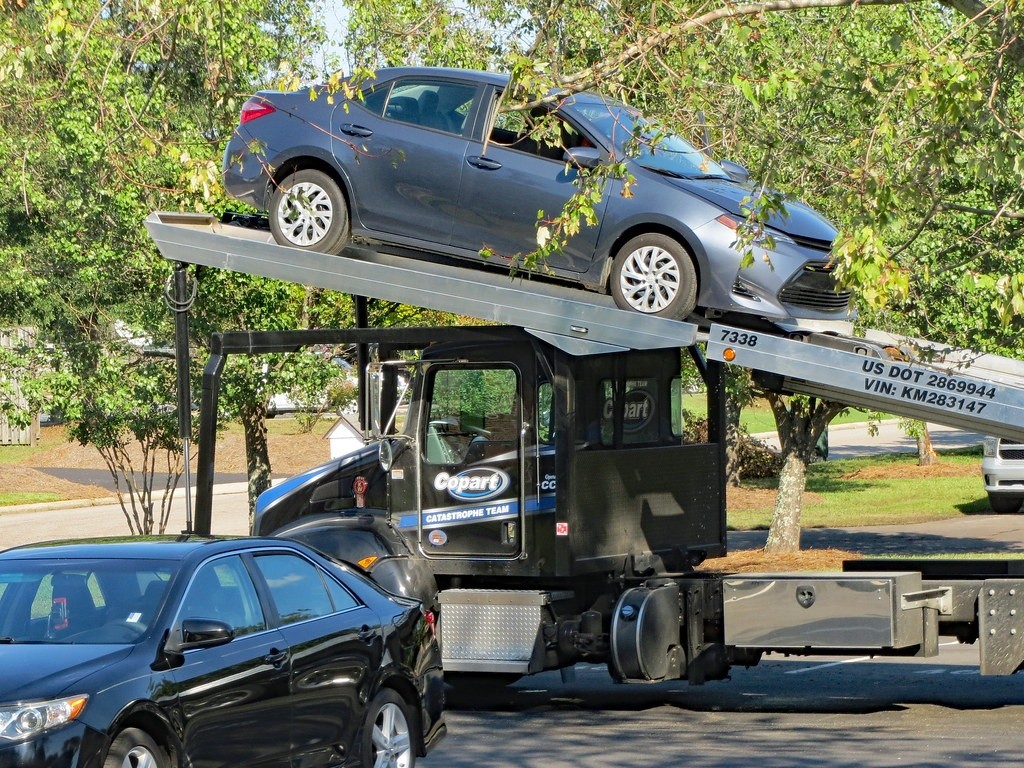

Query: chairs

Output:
49,575,309,631
388,90,585,160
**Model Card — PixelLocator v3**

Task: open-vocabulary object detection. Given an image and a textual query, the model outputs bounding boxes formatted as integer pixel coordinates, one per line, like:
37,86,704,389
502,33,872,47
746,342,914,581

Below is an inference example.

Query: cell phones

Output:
53,598,68,630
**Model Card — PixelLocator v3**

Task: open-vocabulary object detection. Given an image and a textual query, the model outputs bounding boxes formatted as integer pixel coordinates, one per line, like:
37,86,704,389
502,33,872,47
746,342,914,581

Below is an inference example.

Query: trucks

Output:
196,325,1024,711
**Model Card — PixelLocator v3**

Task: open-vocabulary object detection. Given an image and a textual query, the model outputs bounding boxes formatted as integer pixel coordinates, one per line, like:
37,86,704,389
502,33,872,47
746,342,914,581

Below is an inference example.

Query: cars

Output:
982,433,1024,514
225,67,854,319
255,351,361,418
0,535,448,768
